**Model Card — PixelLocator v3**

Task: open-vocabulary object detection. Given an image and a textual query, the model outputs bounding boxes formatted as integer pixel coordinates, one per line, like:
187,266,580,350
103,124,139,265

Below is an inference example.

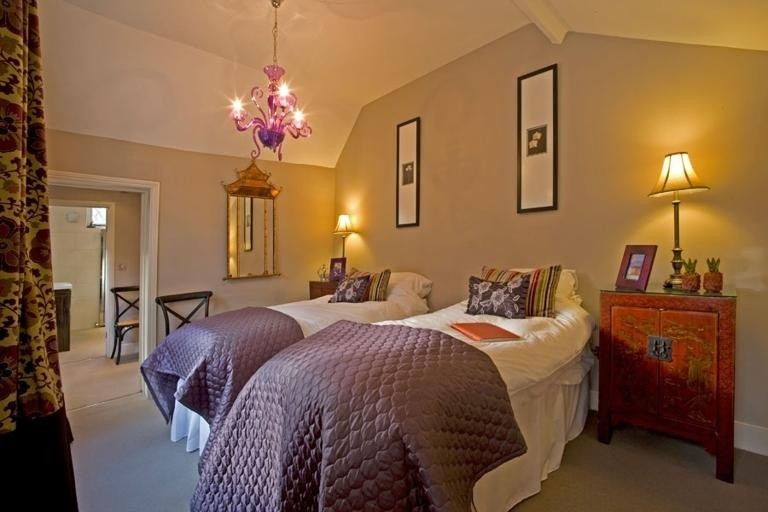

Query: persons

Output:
335,264,341,274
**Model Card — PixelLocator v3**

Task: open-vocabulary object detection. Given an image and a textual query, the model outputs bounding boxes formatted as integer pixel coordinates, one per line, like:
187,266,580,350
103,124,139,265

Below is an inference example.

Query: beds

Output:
140,271,433,455
189,267,599,512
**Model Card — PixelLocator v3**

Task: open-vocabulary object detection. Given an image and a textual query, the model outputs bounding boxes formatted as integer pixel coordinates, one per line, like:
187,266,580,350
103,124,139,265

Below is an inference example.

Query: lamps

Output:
229,0,313,162
331,214,354,258
647,151,710,291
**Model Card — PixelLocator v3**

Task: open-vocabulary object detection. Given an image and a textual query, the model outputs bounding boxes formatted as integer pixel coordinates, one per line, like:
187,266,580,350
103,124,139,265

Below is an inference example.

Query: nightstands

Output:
597,291,736,484
309,281,336,300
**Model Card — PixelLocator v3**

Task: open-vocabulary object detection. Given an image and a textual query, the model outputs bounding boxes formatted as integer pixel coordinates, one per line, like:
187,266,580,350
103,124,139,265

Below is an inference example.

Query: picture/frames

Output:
395,116,420,228
516,63,558,213
329,257,346,282
615,244,657,293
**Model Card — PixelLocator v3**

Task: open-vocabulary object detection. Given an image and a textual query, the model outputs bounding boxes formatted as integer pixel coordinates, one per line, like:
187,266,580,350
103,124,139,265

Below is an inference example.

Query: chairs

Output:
155,290,213,336
110,285,140,364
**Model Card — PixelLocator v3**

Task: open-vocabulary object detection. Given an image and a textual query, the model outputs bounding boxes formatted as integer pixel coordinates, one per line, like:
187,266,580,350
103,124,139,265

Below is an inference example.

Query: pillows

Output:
479,263,562,318
463,274,532,319
344,267,391,301
508,268,584,314
328,274,374,303
388,272,433,299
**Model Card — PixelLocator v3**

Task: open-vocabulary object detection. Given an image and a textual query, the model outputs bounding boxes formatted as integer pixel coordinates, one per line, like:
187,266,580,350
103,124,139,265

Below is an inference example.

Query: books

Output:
451,322,521,342
